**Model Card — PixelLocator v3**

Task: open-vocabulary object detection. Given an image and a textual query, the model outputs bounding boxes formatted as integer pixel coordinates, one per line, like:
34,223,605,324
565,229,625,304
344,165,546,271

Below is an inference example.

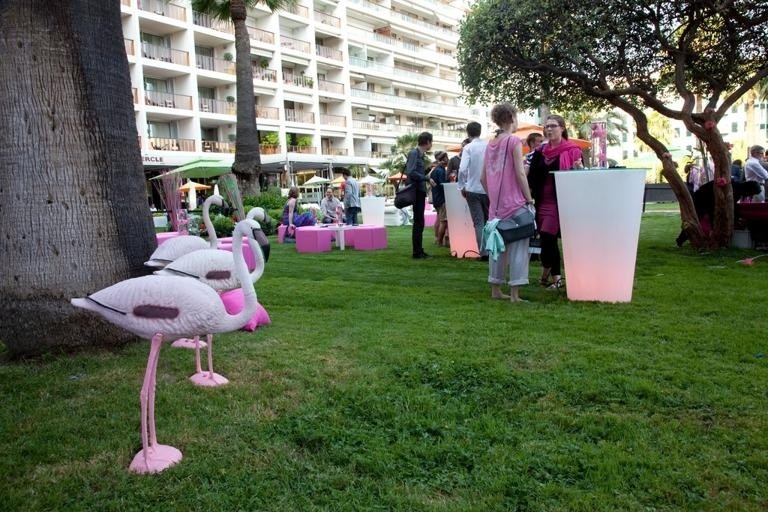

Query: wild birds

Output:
151,207,278,380
72,216,271,469
143,195,237,344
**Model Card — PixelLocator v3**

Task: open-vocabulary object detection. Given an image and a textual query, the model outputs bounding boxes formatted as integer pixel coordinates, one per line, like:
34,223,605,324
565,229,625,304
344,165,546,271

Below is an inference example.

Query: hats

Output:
434,150,447,162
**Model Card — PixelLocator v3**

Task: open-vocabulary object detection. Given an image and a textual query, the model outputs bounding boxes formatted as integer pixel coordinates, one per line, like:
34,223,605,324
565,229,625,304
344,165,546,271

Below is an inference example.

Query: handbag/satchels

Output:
394,182,418,209
496,211,536,245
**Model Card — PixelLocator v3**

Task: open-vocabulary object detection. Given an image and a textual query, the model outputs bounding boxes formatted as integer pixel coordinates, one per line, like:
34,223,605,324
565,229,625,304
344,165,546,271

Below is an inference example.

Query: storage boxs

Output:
734,230,752,251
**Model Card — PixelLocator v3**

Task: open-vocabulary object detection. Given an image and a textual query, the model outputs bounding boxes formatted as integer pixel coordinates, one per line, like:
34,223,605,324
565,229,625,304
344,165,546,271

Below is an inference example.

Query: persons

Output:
684,141,768,236
283,169,361,238
405,103,585,304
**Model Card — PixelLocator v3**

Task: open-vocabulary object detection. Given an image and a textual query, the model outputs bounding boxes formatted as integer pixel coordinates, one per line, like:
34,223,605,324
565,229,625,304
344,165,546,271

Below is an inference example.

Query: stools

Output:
275,214,388,253
385,195,439,228
155,224,272,320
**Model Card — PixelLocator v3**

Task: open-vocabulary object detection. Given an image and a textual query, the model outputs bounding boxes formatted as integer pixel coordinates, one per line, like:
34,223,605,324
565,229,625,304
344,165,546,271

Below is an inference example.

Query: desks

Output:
360,196,386,227
736,200,767,248
548,165,652,306
439,180,485,260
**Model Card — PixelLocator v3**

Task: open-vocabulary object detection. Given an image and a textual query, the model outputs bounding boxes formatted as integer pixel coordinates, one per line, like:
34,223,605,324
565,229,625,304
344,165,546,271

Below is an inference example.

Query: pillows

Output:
219,286,272,336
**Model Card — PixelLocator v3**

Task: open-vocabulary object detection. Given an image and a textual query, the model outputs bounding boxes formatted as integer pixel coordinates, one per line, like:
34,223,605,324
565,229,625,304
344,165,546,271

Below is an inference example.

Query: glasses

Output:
544,124,561,129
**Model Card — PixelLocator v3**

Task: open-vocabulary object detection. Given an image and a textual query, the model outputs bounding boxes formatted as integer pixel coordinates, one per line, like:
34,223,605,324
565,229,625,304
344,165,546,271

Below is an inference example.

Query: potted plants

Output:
260,131,278,154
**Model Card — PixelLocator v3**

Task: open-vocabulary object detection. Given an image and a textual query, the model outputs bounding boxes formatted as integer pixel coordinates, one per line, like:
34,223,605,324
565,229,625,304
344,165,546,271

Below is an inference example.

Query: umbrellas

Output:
360,174,384,185
177,181,211,192
387,171,408,181
